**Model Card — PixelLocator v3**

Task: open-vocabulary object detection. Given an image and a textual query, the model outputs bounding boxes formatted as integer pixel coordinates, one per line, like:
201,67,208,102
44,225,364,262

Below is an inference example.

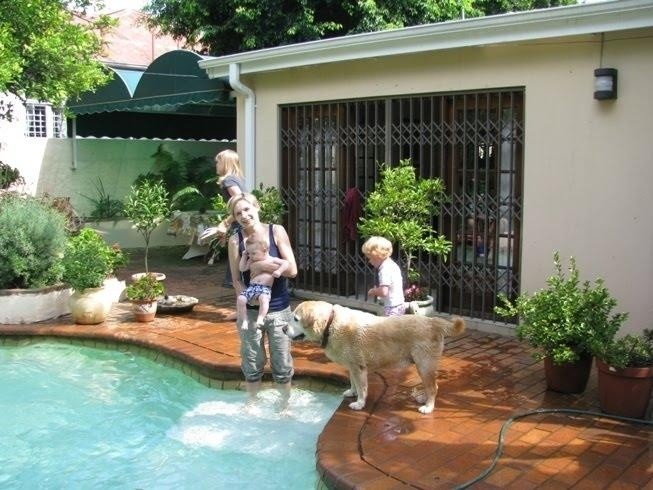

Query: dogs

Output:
282,299,466,416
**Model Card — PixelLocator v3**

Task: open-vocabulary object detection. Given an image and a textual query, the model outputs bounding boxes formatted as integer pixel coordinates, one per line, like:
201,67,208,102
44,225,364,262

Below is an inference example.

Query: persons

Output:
228,192,298,400
362,235,405,318
212,149,252,322
237,232,289,331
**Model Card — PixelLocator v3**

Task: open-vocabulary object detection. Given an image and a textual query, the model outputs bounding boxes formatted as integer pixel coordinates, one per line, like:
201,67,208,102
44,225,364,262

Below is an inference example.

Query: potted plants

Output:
126,272,163,322
494,248,617,394
107,242,120,304
124,179,175,289
357,155,454,317
66,229,110,324
587,313,653,419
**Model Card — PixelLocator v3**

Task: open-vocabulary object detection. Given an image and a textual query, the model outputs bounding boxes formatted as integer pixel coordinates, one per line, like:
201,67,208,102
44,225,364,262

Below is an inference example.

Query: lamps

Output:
594,68,618,101
